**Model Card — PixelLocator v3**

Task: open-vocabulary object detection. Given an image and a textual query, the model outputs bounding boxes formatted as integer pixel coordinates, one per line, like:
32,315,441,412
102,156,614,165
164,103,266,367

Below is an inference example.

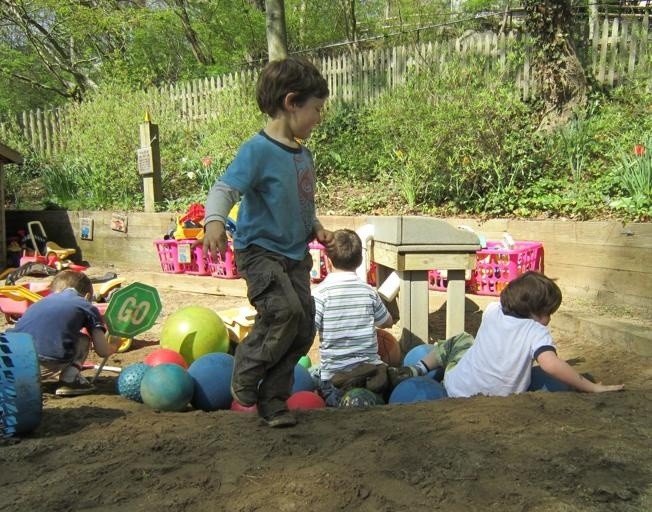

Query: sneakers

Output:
260,409,296,428
366,364,388,390
56,373,96,397
332,363,377,391
388,366,422,387
230,344,258,406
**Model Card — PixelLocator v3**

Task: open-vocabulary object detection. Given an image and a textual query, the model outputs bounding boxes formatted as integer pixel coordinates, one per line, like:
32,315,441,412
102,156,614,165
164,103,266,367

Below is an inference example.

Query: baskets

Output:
309,238,377,286
155,239,239,278
428,240,542,296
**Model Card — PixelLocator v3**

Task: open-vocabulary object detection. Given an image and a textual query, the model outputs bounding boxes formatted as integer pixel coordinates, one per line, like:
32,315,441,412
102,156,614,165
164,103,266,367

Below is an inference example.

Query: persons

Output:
312,229,394,407
388,269,625,399
188,56,336,430
4,272,122,394
